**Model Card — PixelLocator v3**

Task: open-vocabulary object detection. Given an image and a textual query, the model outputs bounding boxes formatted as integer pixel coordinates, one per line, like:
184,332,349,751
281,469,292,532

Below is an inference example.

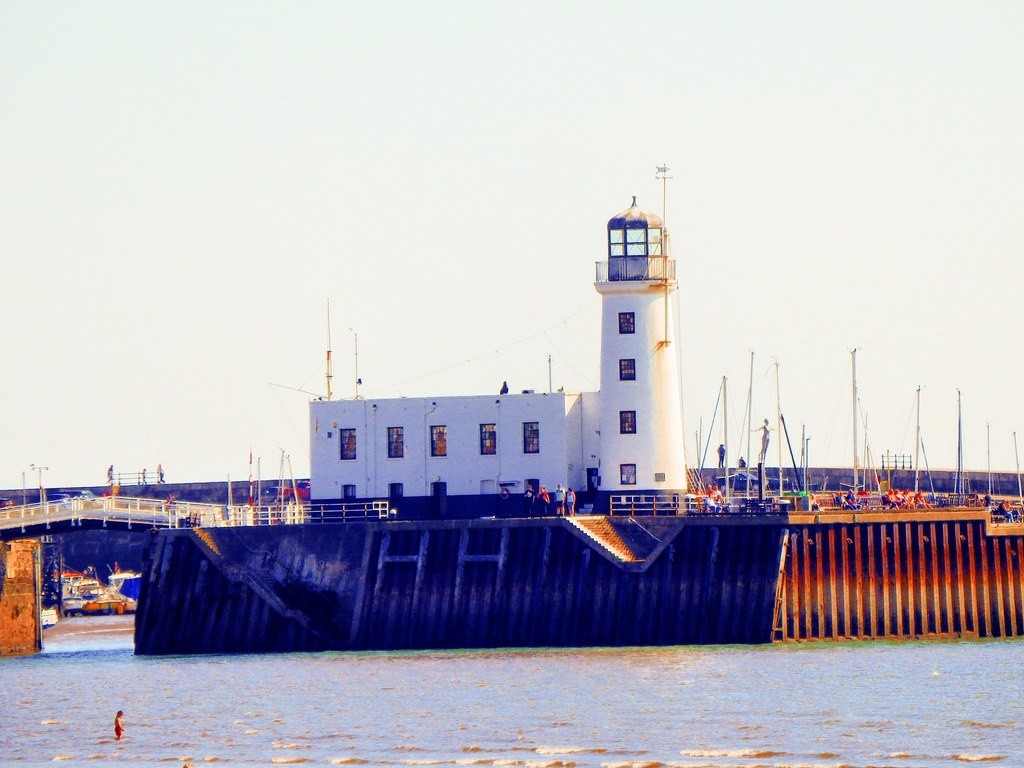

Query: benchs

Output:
824,507,841,511
882,495,903,510
992,515,1004,523
698,497,731,514
928,494,948,508
861,497,885,510
835,497,862,510
967,494,977,507
908,496,935,509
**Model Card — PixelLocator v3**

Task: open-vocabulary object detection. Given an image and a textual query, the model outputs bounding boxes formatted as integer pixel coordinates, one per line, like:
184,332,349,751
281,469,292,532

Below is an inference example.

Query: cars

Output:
279,480,311,503
254,486,290,503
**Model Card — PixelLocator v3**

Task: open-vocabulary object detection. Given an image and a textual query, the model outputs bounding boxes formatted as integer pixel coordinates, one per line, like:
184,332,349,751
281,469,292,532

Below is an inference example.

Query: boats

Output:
59,570,141,616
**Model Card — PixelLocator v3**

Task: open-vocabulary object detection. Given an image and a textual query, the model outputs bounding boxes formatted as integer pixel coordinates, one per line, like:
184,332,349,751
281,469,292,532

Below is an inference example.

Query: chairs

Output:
755,498,773,513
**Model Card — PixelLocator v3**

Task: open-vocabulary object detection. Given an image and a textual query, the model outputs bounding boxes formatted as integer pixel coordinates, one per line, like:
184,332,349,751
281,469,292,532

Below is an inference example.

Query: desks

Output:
783,492,806,511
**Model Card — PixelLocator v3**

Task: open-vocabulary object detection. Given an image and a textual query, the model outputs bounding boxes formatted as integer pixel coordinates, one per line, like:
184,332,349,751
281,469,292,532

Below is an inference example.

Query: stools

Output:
778,499,790,512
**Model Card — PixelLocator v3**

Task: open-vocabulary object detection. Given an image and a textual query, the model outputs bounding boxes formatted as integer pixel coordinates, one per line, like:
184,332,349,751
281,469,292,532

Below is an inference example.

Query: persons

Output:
754,419,777,464
105,465,115,484
537,486,549,517
831,489,862,511
554,483,575,517
969,489,991,511
114,710,124,740
523,484,535,519
157,464,165,484
141,469,147,485
164,494,176,509
738,456,746,468
809,494,823,512
707,485,725,504
886,488,934,510
717,444,725,470
998,499,1021,523
